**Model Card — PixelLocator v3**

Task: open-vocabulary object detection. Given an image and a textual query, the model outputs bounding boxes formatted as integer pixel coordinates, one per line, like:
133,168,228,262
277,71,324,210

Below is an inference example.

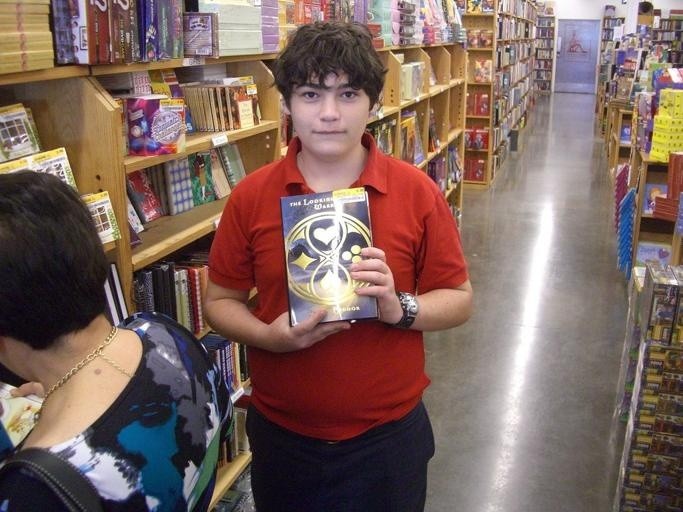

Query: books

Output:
366,60,463,232
280,186,380,327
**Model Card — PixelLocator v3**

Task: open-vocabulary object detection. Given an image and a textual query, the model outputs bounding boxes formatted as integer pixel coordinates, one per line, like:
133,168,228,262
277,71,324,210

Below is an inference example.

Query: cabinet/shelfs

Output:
0,64,123,511
364,0,559,234
90,55,289,512
595,15,682,509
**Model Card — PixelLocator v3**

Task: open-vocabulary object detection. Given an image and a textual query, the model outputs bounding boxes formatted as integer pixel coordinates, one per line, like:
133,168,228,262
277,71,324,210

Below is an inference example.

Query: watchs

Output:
382,290,419,330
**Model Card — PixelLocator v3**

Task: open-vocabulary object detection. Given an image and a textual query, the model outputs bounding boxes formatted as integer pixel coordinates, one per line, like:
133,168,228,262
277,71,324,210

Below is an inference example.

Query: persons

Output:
201,21,474,512
